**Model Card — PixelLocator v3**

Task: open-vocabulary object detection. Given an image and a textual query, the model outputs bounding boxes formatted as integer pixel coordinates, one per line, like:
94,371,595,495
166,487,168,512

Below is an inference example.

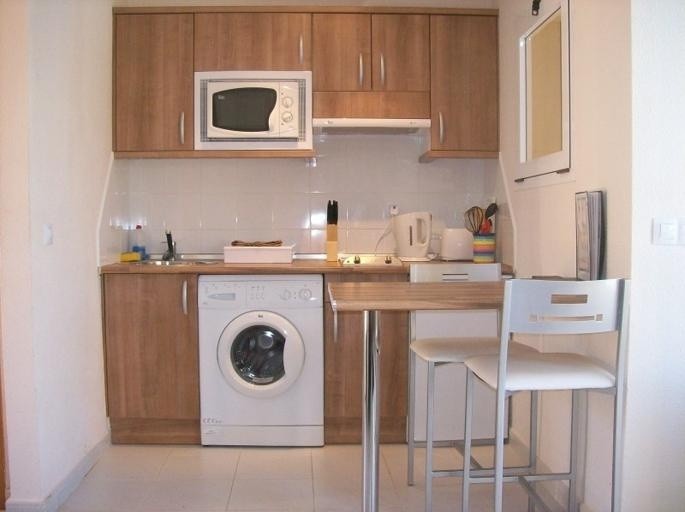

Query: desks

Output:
328,282,588,512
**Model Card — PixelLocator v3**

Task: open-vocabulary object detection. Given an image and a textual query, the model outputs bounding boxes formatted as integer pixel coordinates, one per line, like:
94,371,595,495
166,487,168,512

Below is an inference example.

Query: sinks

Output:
142,258,222,268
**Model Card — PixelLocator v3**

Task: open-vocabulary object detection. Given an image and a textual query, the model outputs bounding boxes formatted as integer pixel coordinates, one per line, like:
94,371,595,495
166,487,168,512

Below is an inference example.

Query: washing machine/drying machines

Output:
196,274,326,447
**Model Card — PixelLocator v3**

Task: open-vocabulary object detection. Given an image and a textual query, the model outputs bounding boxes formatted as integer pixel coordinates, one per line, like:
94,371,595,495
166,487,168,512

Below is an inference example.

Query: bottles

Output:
132,225,146,259
474,233,496,262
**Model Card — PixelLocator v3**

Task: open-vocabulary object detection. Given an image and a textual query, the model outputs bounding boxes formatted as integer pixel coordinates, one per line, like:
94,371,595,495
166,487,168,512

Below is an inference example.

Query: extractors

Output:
311,118,432,129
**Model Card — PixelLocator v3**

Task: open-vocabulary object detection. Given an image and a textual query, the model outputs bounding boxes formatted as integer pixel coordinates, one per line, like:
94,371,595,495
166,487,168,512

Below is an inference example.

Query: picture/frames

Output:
512,0,569,181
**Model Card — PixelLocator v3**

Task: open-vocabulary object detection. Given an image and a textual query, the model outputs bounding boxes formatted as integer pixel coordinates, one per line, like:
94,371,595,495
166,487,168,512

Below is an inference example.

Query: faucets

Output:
159,229,177,261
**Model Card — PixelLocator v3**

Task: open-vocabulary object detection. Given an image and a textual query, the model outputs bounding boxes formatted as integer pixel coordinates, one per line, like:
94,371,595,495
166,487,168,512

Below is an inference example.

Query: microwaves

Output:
195,71,313,151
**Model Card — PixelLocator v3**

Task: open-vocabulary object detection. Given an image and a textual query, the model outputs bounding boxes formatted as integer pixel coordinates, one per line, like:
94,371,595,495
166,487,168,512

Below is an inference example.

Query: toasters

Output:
440,228,473,261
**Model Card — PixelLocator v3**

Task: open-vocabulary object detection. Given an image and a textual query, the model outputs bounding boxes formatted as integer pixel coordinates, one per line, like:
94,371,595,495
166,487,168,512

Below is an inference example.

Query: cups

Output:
160,242,176,261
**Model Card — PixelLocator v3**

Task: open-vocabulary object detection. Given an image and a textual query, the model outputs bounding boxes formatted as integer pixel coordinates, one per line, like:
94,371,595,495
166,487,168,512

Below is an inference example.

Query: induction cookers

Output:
339,254,400,265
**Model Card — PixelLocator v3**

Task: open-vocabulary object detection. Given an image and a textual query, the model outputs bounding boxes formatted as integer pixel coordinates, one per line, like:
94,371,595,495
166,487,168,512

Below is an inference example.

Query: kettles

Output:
392,211,433,262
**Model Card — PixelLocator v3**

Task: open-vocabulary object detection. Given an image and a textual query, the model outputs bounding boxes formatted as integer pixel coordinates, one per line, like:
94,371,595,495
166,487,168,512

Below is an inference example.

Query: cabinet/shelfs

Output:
194,6,315,76
111,5,194,160
315,9,432,90
323,262,409,445
100,261,202,445
312,93,431,132
420,8,499,161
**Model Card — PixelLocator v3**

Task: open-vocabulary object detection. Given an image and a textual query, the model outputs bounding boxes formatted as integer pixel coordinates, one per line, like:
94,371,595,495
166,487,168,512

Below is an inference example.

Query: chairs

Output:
463,279,628,512
407,263,538,488
